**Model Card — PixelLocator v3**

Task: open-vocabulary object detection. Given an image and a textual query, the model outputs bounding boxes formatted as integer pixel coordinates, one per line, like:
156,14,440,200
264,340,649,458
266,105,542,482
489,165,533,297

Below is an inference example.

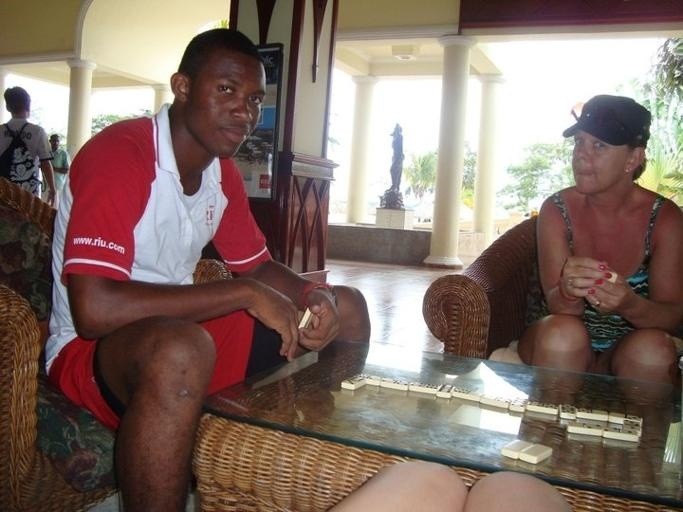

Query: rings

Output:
565,276,575,289
592,300,602,308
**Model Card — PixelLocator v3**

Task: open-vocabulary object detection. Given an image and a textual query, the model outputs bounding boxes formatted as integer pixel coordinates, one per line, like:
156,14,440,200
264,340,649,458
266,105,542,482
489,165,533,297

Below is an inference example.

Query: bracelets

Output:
301,281,338,313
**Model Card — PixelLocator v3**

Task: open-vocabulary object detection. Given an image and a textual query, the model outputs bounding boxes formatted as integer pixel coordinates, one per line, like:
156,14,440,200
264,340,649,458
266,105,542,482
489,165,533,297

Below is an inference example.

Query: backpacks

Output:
0,123,43,200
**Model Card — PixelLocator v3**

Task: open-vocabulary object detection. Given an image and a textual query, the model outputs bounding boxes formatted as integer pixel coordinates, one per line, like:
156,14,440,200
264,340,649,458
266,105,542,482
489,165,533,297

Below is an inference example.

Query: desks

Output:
193,342,683,511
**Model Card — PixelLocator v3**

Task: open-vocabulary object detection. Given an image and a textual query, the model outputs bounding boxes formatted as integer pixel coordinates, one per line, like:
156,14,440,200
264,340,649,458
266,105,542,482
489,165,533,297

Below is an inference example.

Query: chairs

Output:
422,216,683,425
0,175,235,512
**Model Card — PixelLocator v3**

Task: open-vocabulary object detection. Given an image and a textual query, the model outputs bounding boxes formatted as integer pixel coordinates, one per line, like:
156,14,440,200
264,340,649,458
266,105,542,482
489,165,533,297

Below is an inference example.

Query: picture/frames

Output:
225,43,283,203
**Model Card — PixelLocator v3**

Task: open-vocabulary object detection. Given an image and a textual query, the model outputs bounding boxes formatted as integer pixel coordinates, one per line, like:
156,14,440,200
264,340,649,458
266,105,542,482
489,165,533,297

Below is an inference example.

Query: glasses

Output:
571,101,646,146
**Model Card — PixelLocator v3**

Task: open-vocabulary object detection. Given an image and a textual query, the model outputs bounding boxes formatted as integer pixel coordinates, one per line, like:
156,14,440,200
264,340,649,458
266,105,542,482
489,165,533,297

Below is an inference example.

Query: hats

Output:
562,95,652,146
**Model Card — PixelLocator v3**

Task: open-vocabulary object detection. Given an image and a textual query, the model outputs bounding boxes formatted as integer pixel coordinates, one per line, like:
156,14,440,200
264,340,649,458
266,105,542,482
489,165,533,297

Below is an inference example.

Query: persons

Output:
324,457,580,512
515,93,680,407
43,26,371,511
42,134,71,208
0,87,56,209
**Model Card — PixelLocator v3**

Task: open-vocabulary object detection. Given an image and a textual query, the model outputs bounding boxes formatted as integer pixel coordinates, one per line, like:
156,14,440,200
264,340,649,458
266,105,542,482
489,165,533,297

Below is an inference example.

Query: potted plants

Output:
375,190,417,231
